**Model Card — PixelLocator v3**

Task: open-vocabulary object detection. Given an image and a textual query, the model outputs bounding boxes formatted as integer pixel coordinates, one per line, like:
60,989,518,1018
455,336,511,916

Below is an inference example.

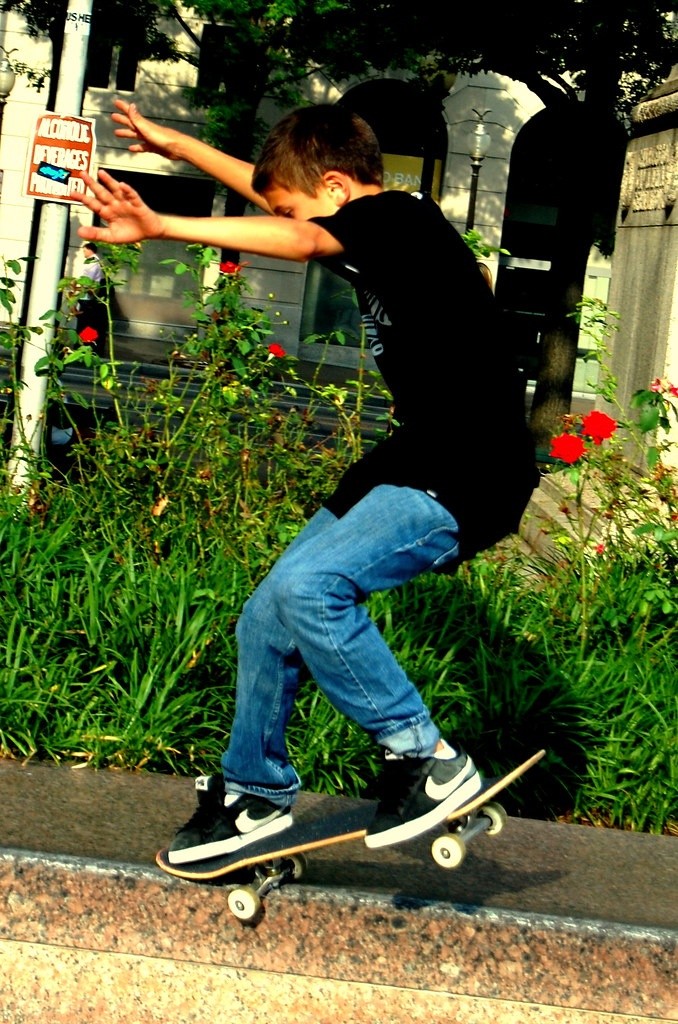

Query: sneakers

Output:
364,741,482,849
168,772,294,866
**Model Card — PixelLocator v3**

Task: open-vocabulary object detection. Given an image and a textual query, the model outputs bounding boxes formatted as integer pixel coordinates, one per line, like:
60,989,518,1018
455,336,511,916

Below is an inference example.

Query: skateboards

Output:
153,745,551,920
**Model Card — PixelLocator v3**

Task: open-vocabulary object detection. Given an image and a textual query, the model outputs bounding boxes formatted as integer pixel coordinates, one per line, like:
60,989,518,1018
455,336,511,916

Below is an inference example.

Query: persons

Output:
68,243,107,347
67,95,538,865
